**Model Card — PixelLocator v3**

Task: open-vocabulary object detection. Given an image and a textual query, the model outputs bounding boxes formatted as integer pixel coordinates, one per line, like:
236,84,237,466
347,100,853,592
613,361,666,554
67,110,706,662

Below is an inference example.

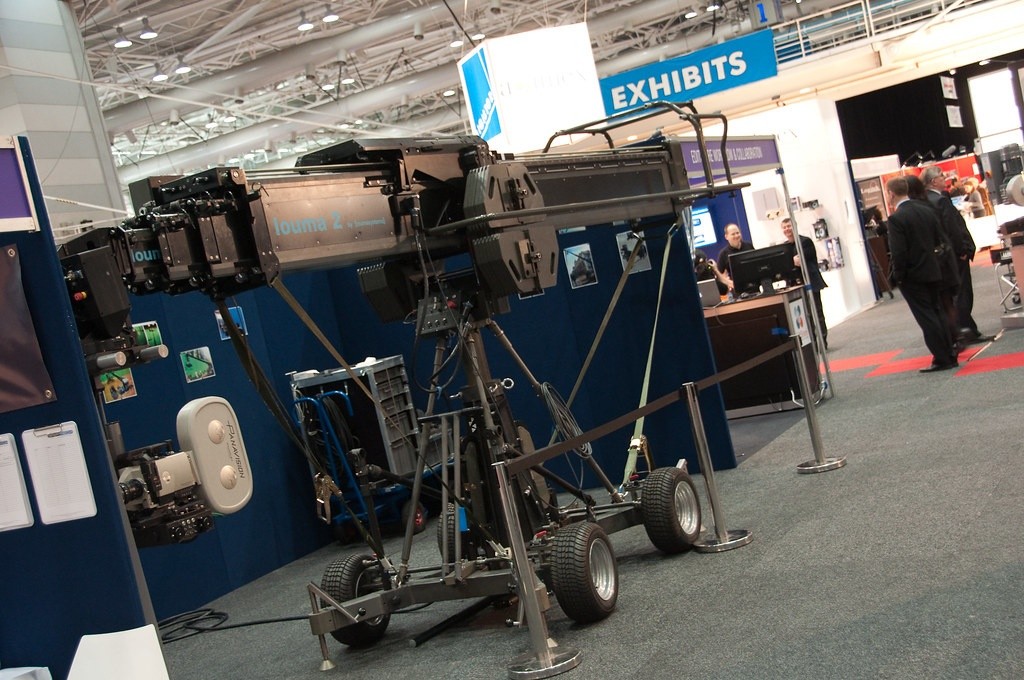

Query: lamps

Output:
152,62,168,81
175,54,191,73
206,115,217,128
224,110,236,122
340,68,354,84
114,25,133,48
139,16,158,39
471,24,486,40
298,10,314,32
450,29,463,47
322,3,339,22
322,74,335,90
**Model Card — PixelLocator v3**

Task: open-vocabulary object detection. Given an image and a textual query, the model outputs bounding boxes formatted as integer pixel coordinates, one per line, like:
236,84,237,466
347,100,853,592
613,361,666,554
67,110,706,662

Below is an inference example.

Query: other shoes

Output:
920,363,952,372
952,361,958,367
824,342,827,349
953,341,964,352
967,334,996,344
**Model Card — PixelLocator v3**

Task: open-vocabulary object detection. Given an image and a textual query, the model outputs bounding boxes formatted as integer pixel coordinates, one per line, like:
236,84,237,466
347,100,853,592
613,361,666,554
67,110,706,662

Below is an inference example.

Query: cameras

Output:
696,262,709,274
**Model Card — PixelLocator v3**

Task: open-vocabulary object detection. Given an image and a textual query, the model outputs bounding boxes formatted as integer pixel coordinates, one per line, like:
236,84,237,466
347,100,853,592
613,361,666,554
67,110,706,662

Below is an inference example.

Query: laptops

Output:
697,279,721,307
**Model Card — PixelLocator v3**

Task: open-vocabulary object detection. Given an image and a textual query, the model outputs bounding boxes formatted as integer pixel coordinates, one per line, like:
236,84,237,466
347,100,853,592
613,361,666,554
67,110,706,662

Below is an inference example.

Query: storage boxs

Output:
293,356,451,478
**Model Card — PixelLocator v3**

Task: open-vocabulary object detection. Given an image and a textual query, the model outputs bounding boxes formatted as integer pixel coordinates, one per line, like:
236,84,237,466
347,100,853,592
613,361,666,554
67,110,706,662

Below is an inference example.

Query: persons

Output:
695,258,728,295
781,218,828,353
885,175,960,373
949,178,963,198
716,223,754,288
919,166,996,344
964,178,991,252
872,210,888,236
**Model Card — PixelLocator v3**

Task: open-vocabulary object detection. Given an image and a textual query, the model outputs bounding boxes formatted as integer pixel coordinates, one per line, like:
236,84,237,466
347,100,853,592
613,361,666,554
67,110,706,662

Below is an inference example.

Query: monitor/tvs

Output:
728,241,800,298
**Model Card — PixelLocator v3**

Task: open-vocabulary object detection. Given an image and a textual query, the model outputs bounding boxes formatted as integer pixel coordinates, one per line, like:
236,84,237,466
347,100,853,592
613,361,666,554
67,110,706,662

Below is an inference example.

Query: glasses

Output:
930,174,944,181
